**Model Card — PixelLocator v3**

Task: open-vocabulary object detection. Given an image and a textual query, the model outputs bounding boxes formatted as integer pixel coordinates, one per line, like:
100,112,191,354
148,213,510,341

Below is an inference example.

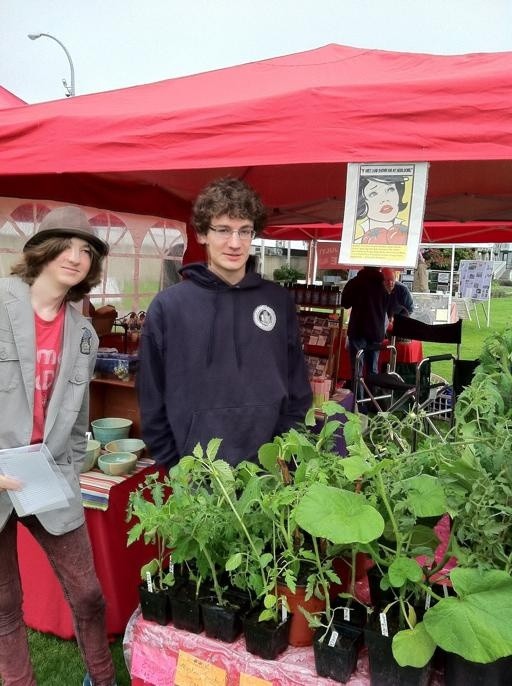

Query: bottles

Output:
283,281,342,309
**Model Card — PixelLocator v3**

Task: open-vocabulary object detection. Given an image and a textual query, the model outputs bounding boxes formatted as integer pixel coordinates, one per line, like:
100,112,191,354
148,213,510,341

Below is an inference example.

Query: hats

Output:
22,206,110,258
383,267,395,279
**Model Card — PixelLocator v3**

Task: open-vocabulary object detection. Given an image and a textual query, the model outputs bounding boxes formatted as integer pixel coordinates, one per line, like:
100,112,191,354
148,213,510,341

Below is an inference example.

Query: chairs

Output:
352,312,462,451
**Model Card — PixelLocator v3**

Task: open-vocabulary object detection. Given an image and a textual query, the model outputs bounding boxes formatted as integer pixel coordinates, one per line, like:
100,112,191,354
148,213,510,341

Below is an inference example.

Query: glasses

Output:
209,225,256,240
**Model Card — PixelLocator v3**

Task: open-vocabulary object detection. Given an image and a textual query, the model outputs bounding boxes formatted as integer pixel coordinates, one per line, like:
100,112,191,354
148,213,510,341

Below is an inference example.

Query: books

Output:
304,353,332,400
296,314,332,352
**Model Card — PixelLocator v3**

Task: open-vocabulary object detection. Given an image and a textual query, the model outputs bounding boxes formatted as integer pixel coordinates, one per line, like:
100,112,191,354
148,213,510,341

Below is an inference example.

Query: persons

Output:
354,175,409,244
0,205,118,686
342,266,402,415
133,175,312,488
379,267,416,338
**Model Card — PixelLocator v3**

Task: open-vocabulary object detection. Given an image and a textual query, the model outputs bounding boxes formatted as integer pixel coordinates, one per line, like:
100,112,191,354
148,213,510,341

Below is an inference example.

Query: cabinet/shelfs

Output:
284,283,345,398
16,332,171,643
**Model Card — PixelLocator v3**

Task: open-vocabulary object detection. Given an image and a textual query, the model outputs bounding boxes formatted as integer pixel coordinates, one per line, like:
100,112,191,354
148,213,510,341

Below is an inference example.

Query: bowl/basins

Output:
79,440,102,474
97,452,138,476
90,417,133,450
104,437,146,459
